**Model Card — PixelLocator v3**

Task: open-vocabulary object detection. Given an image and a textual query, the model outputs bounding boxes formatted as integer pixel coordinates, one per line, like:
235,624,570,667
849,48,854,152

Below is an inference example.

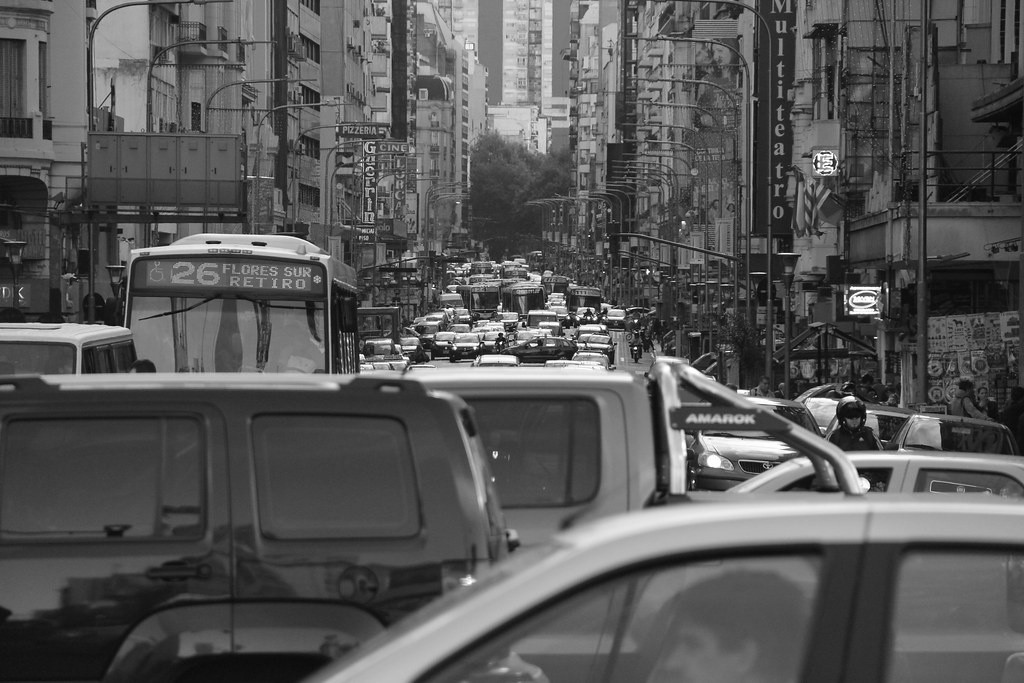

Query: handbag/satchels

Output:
961,396,983,418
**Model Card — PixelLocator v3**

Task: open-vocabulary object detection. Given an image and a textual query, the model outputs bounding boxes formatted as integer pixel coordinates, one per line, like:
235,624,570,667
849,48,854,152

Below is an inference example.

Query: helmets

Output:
836,396,866,418
634,332,639,335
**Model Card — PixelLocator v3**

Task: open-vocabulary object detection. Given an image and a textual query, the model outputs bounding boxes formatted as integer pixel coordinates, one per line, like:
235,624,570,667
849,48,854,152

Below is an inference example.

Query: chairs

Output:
638,571,812,683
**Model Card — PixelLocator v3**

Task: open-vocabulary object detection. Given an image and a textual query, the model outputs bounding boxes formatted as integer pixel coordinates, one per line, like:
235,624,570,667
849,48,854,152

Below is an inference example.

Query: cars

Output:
0,250,1024,683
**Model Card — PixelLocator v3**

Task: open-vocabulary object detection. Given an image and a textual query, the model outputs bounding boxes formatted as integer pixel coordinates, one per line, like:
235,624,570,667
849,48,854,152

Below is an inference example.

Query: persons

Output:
496,333,505,352
602,308,671,358
707,199,736,225
583,308,593,318
749,364,1024,452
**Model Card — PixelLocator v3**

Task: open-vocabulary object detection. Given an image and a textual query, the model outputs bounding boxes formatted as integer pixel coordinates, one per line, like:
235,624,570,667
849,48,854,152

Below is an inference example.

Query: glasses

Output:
846,411,861,419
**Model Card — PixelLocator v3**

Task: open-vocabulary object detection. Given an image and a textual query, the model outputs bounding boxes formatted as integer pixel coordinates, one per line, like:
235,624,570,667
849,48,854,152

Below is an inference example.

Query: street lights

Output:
83,1,778,402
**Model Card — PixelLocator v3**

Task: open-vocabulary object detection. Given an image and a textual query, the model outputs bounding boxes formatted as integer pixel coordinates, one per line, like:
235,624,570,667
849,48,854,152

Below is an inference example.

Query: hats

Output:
70,277,78,284
498,333,503,336
1011,386,1024,400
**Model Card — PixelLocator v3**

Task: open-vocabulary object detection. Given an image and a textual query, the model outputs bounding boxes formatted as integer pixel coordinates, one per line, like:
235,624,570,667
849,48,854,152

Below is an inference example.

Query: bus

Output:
109,232,361,377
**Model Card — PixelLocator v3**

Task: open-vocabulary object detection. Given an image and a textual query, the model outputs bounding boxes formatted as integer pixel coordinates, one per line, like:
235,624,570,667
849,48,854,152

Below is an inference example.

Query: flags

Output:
791,167,830,239
